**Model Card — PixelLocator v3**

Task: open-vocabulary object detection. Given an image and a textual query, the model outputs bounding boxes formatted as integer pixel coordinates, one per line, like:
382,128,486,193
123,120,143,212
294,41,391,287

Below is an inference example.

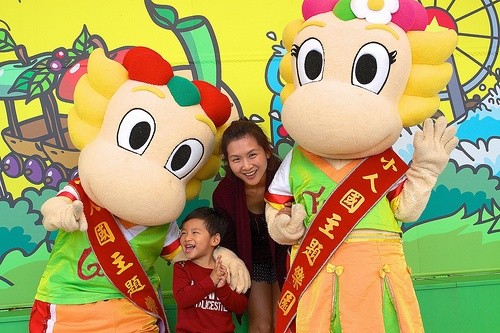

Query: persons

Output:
213,117,292,333
173,206,247,333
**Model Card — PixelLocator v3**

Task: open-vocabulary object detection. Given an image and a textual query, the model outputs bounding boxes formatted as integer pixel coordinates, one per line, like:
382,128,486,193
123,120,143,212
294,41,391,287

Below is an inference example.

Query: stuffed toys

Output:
29,47,251,333
263,0,459,333
